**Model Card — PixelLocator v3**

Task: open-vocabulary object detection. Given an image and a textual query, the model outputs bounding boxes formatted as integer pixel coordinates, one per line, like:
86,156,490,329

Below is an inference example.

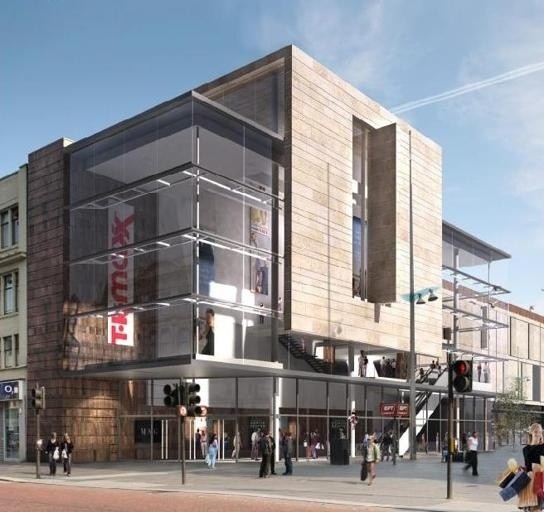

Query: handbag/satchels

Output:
52,447,60,460
361,462,367,480
498,455,544,508
205,454,209,464
62,448,68,459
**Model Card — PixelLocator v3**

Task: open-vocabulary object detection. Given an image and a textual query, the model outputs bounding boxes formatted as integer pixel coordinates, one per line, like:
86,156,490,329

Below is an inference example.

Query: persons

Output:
435,360,441,368
429,360,434,369
462,431,479,476
515,423,544,512
43,432,60,475
477,361,482,381
418,367,424,378
195,426,398,486
199,308,213,355
482,361,490,382
59,432,73,476
355,348,397,378
